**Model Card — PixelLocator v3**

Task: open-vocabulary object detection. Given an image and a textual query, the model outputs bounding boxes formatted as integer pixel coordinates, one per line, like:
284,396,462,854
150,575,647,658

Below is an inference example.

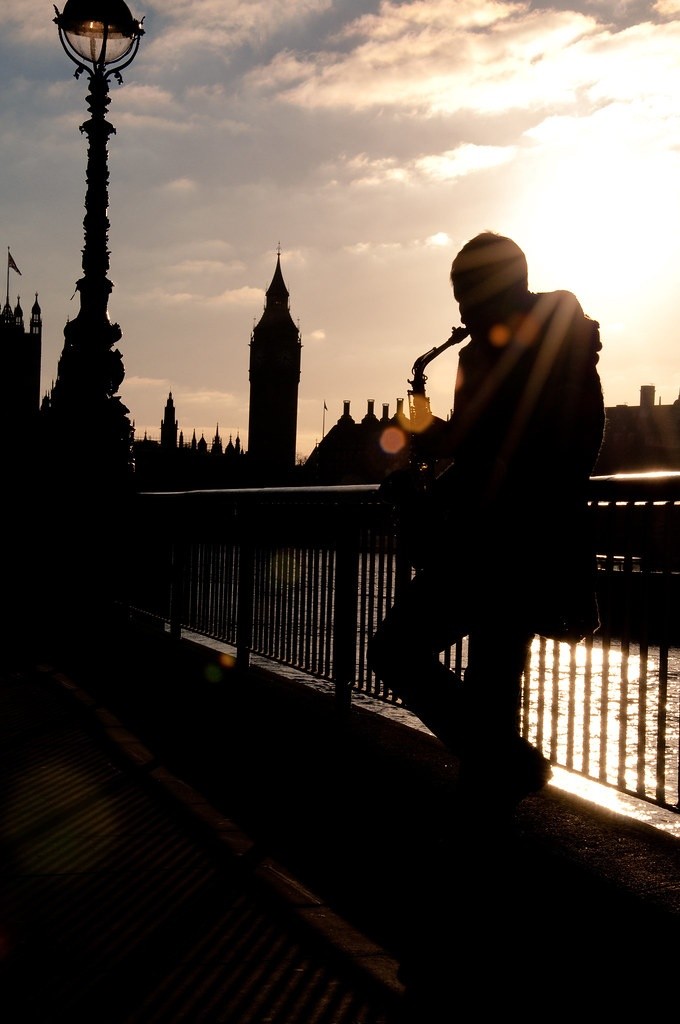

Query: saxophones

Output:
371,327,479,586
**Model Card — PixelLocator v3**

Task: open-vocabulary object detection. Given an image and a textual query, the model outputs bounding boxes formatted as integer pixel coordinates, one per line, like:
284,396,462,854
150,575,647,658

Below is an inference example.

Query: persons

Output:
365,232,607,802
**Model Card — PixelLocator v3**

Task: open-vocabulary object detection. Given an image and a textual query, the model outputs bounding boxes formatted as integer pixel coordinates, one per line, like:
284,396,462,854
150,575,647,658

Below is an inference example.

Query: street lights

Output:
53,0,146,348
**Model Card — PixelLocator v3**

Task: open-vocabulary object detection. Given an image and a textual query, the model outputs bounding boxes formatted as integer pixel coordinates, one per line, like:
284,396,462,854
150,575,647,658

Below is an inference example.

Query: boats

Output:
596,555,680,592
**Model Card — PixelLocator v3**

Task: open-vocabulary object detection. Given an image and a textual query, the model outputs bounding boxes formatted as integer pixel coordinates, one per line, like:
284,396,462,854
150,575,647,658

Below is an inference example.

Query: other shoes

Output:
426,739,544,828
